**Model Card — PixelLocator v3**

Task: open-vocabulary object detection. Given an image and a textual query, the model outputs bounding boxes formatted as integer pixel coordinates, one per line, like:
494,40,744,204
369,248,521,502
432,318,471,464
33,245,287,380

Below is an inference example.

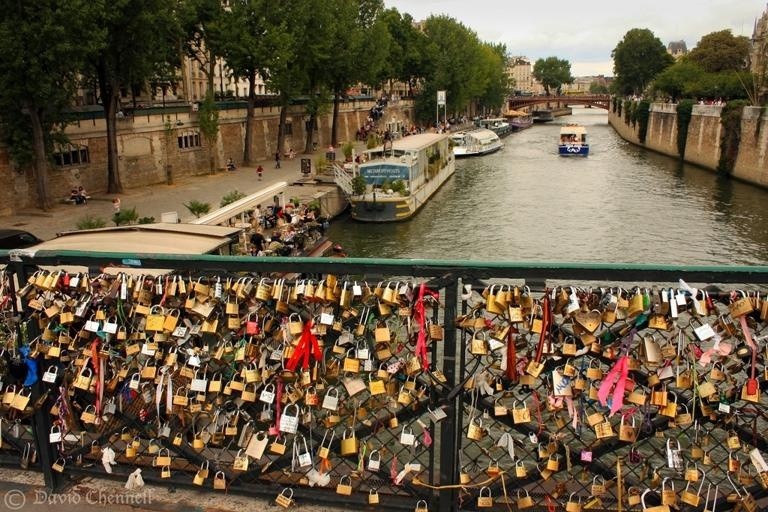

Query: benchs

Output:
63,196,91,204
218,166,231,171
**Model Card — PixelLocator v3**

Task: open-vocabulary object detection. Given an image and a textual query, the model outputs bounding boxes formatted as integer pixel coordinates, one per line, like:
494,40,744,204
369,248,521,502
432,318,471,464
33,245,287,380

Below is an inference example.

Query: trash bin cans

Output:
326,151,335,163
301,158,311,177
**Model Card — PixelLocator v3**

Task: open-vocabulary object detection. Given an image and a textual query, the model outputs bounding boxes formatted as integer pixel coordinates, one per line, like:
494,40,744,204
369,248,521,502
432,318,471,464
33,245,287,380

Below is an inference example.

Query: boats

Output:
479,117,512,137
448,127,504,156
350,133,455,222
532,110,553,122
503,110,533,130
558,124,589,157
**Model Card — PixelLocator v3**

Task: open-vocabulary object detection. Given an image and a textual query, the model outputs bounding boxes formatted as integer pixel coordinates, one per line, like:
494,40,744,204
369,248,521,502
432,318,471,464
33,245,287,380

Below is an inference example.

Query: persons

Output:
328,144,333,152
275,151,281,168
112,197,120,213
401,124,426,137
432,112,488,134
227,158,235,170
71,190,82,204
256,165,264,181
289,149,296,160
249,198,330,258
494,121,498,127
625,94,727,106
358,95,389,140
78,186,88,204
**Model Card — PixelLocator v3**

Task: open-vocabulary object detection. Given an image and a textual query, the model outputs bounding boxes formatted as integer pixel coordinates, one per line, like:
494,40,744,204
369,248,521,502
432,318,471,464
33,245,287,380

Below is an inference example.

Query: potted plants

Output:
341,141,354,162
